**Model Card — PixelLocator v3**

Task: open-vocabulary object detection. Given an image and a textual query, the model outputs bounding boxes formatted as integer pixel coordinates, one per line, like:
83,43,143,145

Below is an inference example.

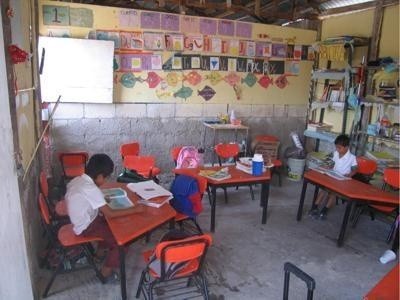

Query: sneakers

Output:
310,205,329,218
92,253,121,283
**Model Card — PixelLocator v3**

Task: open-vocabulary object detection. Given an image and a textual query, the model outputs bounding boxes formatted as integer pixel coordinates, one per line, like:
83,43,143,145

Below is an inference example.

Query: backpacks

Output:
149,230,200,277
177,146,201,169
169,175,202,215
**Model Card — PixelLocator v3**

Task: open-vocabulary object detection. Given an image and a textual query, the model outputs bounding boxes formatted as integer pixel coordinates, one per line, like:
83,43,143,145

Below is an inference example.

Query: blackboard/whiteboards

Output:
37,36,115,103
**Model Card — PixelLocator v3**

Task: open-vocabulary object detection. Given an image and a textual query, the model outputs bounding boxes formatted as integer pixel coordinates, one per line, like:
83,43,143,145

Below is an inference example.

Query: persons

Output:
63,154,128,285
304,135,359,220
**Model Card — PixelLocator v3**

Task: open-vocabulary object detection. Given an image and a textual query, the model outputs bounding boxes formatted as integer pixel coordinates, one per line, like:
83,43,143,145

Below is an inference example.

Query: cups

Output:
379,250,396,264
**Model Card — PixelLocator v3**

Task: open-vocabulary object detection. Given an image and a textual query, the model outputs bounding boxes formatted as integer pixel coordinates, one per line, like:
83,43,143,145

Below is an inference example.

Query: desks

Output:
296,164,400,248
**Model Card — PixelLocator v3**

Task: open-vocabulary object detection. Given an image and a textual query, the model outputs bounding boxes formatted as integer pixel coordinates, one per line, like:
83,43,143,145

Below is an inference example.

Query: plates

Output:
263,164,274,168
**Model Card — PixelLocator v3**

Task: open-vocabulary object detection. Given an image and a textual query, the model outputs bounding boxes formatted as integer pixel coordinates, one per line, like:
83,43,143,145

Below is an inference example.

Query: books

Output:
101,187,134,212
127,180,173,200
307,84,400,176
312,167,353,181
136,195,172,208
236,157,267,175
199,169,232,182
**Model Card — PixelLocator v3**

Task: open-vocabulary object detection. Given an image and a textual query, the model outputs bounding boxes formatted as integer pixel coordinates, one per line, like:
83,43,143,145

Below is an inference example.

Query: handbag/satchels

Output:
352,172,374,184
118,168,159,184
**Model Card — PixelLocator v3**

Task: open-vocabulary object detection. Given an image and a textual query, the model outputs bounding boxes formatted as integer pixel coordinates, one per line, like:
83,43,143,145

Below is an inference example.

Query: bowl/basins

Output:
231,118,241,124
367,121,400,141
239,157,252,163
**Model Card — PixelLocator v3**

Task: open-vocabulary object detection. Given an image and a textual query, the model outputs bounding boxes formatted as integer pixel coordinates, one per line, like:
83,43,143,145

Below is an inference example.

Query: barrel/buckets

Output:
286,158,306,182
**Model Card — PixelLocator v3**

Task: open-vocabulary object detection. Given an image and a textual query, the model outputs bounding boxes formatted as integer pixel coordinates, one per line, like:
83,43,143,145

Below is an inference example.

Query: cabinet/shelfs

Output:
202,122,250,167
303,35,399,151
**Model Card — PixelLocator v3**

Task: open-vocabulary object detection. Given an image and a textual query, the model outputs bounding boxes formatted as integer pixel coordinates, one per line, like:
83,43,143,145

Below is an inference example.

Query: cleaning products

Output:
230,110,236,123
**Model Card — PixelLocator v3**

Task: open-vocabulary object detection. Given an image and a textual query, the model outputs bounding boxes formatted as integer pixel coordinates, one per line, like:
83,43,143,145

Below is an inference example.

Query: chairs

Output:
336,156,399,239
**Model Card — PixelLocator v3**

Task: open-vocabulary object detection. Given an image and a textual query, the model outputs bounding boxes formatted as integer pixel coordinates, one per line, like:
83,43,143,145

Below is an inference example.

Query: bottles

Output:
252,154,264,176
327,59,331,70
223,110,235,123
